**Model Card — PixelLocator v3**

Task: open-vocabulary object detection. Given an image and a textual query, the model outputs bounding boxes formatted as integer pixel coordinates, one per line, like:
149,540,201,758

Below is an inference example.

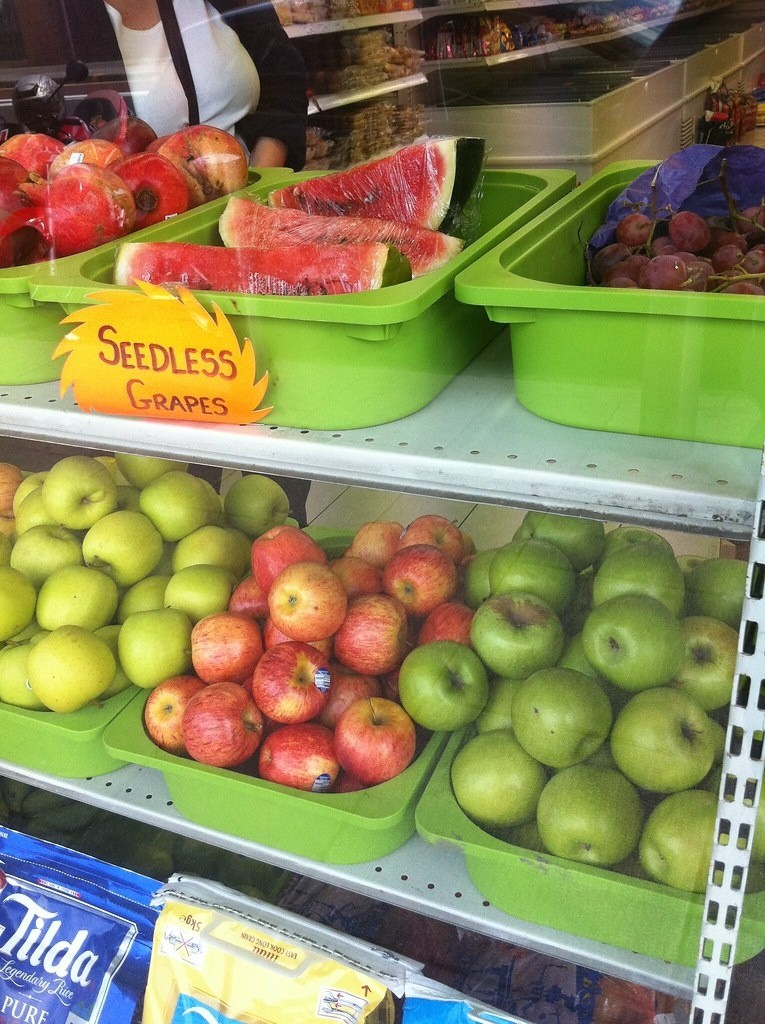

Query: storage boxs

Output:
1,158,765,454
1,516,765,969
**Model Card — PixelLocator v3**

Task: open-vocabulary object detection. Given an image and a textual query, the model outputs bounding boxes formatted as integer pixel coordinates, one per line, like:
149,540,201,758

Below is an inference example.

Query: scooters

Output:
0,62,131,152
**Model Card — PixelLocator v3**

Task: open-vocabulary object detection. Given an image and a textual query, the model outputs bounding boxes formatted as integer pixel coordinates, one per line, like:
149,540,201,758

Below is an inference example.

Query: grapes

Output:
593,206,765,299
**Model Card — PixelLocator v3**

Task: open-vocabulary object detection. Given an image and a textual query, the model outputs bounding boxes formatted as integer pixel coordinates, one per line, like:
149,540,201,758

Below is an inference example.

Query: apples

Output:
0,450,290,713
145,513,481,793
400,511,764,902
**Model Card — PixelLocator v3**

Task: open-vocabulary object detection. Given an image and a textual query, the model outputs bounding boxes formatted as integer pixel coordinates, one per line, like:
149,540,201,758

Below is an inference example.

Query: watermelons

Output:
109,137,486,295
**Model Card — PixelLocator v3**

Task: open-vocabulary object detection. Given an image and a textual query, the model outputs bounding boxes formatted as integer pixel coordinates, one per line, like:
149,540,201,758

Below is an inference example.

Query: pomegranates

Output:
0,124,247,271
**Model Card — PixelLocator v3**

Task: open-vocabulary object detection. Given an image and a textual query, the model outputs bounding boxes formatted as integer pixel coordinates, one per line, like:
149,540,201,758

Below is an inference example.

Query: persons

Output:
58,0,309,175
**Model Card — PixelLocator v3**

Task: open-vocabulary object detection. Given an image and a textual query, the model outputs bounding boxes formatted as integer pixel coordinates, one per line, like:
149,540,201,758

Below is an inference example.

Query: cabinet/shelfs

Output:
279,0,742,115
1,320,765,1024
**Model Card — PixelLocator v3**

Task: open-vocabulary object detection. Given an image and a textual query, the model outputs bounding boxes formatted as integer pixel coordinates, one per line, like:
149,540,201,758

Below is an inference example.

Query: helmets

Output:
12,75,67,128
